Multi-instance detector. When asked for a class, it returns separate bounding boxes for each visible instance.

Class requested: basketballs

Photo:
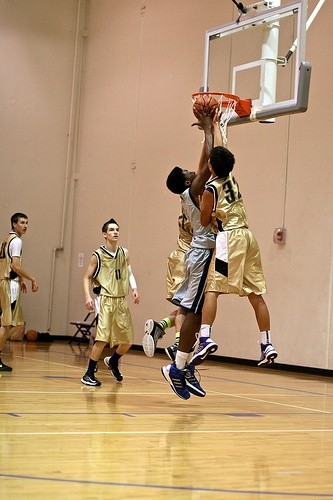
[192,96,220,121]
[27,329,38,342]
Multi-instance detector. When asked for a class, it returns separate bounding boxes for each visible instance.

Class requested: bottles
[89,333,94,347]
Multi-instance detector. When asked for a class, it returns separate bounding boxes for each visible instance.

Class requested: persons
[188,110,277,366]
[81,218,140,386]
[142,207,193,362]
[161,107,224,400]
[0,213,39,372]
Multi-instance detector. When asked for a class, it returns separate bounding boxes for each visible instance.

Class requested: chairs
[68,312,98,346]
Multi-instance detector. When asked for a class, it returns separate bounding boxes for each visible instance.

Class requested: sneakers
[142,319,165,357]
[184,364,206,397]
[0,361,12,371]
[258,343,278,366]
[161,361,190,400]
[189,333,218,366]
[81,364,101,386]
[104,357,123,381]
[165,343,178,363]
